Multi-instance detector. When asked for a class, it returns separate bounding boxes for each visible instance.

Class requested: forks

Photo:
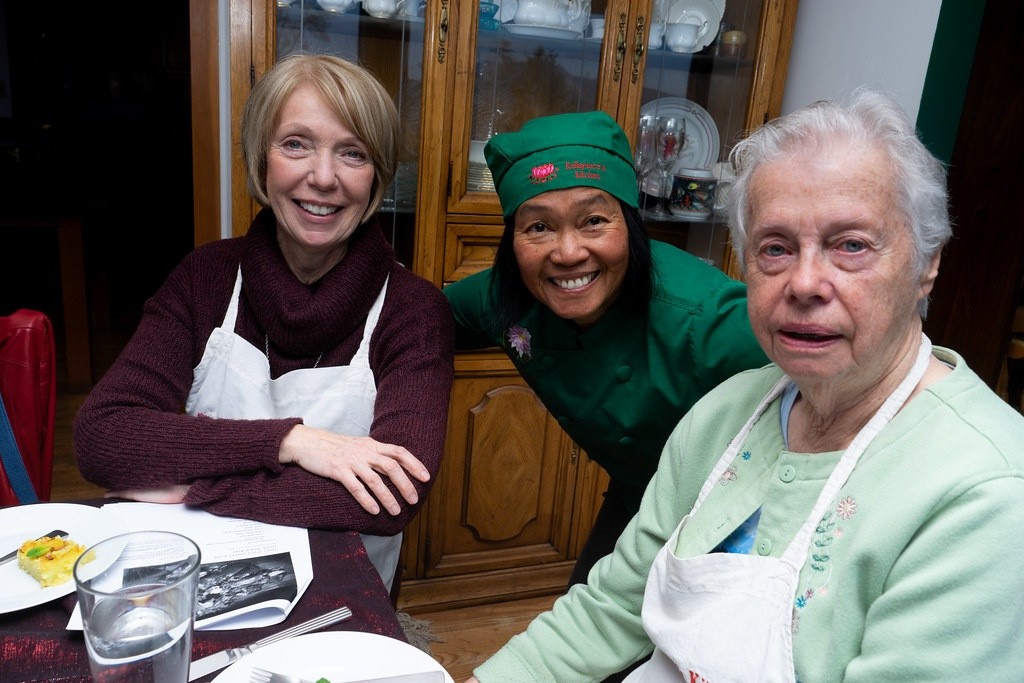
[249,665,445,683]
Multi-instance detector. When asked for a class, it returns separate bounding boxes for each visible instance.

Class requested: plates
[210,631,455,683]
[638,96,721,197]
[502,24,582,41]
[467,161,497,193]
[0,503,129,614]
[480,0,518,24]
[668,0,720,53]
[395,15,425,23]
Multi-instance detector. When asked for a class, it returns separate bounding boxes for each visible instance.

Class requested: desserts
[16,534,96,589]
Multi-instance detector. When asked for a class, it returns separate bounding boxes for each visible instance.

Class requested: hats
[483,110,641,217]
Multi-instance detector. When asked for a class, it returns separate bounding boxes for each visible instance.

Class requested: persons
[438,105,769,593]
[442,92,1024,682]
[69,52,455,598]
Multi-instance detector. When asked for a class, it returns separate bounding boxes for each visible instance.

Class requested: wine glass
[637,118,660,197]
[648,117,686,221]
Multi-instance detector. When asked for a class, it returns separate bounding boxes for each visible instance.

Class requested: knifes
[188,607,352,682]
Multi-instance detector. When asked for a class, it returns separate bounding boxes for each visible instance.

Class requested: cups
[647,23,666,50]
[668,167,732,222]
[361,0,403,19]
[469,141,488,163]
[316,0,355,15]
[397,0,426,16]
[72,531,202,683]
[276,0,297,7]
[667,20,711,53]
[590,19,604,38]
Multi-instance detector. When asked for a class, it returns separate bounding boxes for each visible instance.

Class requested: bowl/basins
[480,2,500,18]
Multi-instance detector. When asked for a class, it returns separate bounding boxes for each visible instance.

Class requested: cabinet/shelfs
[441,0,800,288]
[414,355,612,614]
[190,1,457,621]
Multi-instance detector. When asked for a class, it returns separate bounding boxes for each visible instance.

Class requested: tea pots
[514,0,581,29]
